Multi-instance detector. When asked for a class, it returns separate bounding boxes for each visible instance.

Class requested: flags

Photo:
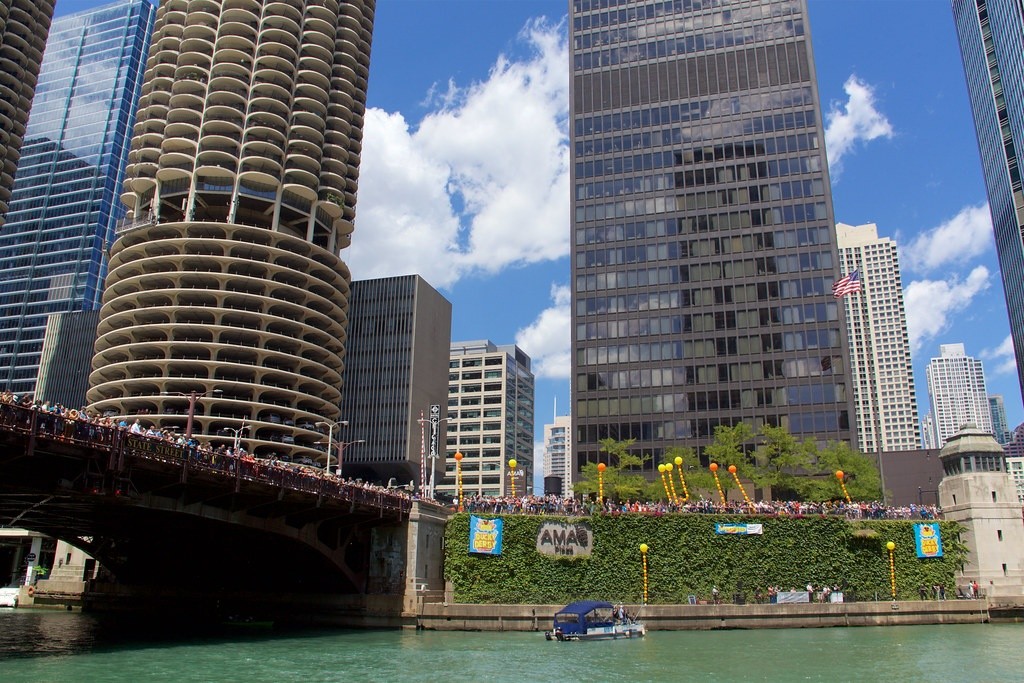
[832,269,861,299]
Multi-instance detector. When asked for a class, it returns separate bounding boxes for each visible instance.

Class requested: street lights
[314,440,366,479]
[224,425,252,455]
[160,390,223,445]
[417,417,452,502]
[314,421,349,478]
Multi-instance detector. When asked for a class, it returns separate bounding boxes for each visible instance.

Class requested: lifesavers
[28,585,34,595]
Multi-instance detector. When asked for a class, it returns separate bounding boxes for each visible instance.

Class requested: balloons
[887,541,896,597]
[508,459,517,495]
[455,452,462,511]
[729,465,750,502]
[598,463,605,499]
[709,462,725,502]
[640,543,648,601]
[658,456,689,501]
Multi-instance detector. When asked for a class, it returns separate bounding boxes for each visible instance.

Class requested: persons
[453,496,943,520]
[614,606,628,620]
[0,388,436,506]
[969,580,979,598]
[958,585,964,596]
[754,584,782,604]
[790,587,796,592]
[713,587,719,605]
[919,583,945,600]
[806,582,841,603]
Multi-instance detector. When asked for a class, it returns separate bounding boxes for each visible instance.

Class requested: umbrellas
[836,471,852,504]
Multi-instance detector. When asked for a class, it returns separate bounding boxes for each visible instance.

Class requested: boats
[545,601,647,642]
